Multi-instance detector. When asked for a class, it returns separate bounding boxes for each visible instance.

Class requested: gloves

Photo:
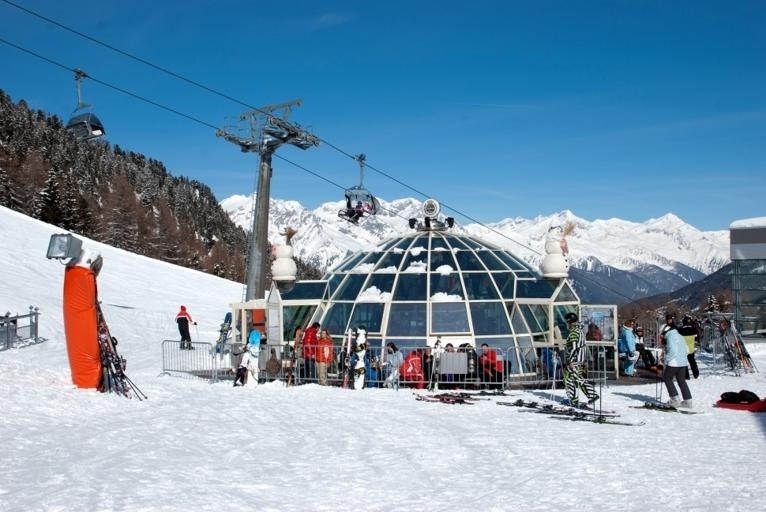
[194,322,197,325]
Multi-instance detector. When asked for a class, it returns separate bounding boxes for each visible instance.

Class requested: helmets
[683,318,690,325]
[635,327,644,336]
[565,313,578,323]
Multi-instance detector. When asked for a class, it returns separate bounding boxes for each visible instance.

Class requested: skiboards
[412,390,523,404]
[339,215,359,226]
[426,335,441,392]
[286,329,301,387]
[343,328,352,389]
[627,402,705,414]
[720,320,759,376]
[97,299,132,399]
[496,398,645,426]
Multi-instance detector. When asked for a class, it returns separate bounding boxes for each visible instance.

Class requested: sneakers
[680,399,692,406]
[667,395,679,405]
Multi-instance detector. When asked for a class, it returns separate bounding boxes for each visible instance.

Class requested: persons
[587,310,700,406]
[351,203,372,220]
[232,321,504,387]
[174,305,197,350]
[557,312,599,408]
[346,200,363,217]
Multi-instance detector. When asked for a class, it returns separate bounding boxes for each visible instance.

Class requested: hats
[181,306,186,310]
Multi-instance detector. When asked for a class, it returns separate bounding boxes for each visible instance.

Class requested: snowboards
[216,312,232,353]
[233,329,260,385]
[353,325,368,390]
